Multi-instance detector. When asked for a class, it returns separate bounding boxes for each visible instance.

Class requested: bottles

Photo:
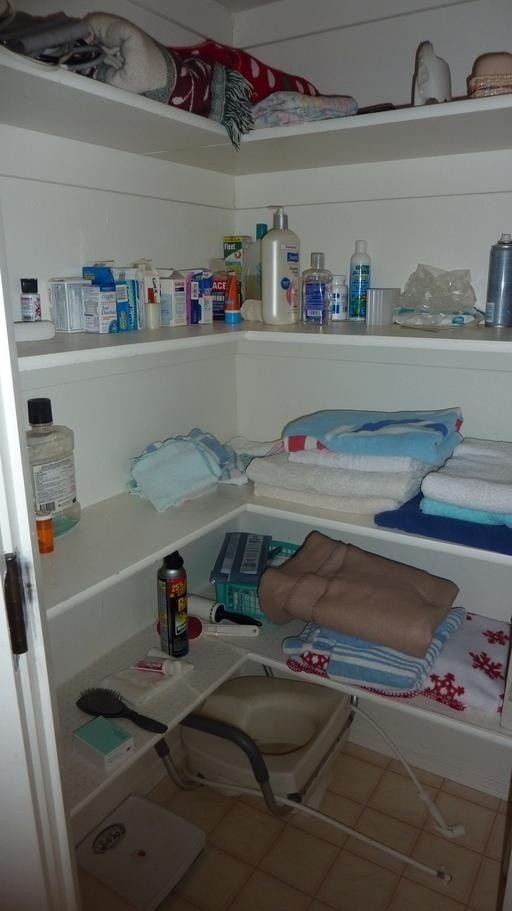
[347,240,372,322]
[22,398,81,538]
[298,252,333,327]
[260,206,304,327]
[483,233,512,328]
[157,550,189,658]
[331,275,349,322]
[241,223,269,300]
[34,512,56,553]
[20,279,41,321]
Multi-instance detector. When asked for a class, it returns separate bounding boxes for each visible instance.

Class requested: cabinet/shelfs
[0,0,512,857]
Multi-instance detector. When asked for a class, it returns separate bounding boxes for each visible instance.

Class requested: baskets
[215,541,301,622]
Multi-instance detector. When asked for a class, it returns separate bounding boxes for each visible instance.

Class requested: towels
[124,402,512,556]
[256,527,512,718]
